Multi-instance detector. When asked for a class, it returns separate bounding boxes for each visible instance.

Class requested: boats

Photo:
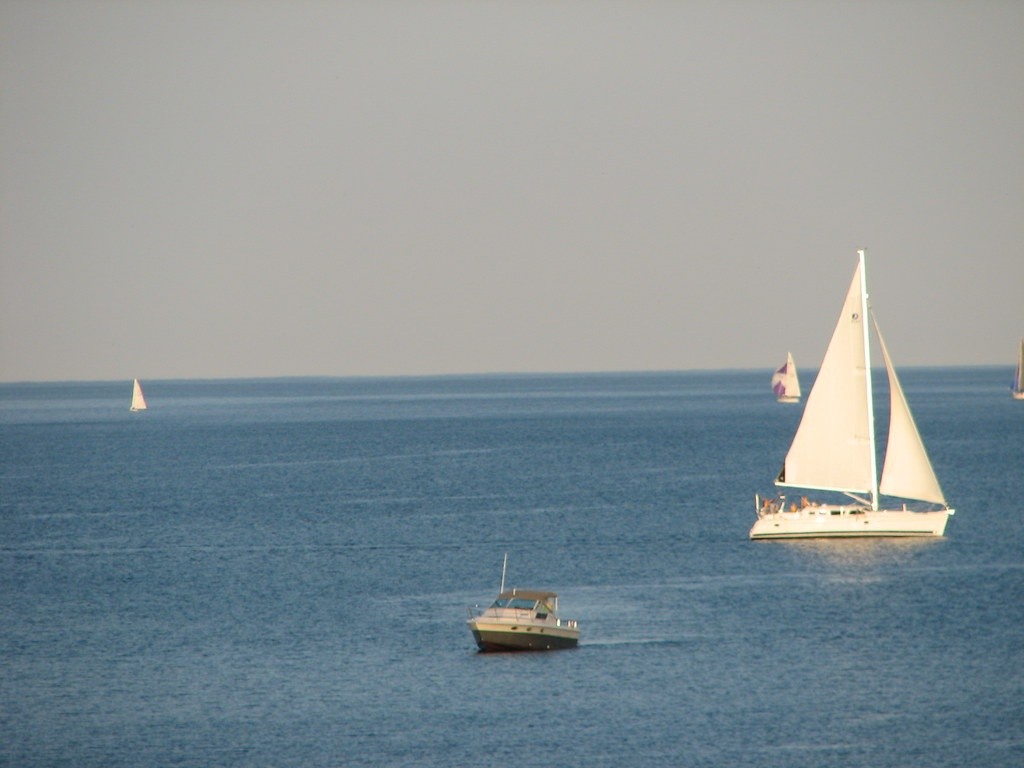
[464,551,582,652]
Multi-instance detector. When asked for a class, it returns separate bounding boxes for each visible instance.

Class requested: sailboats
[1011,337,1024,399]
[128,377,146,412]
[747,250,957,540]
[768,352,805,405]
